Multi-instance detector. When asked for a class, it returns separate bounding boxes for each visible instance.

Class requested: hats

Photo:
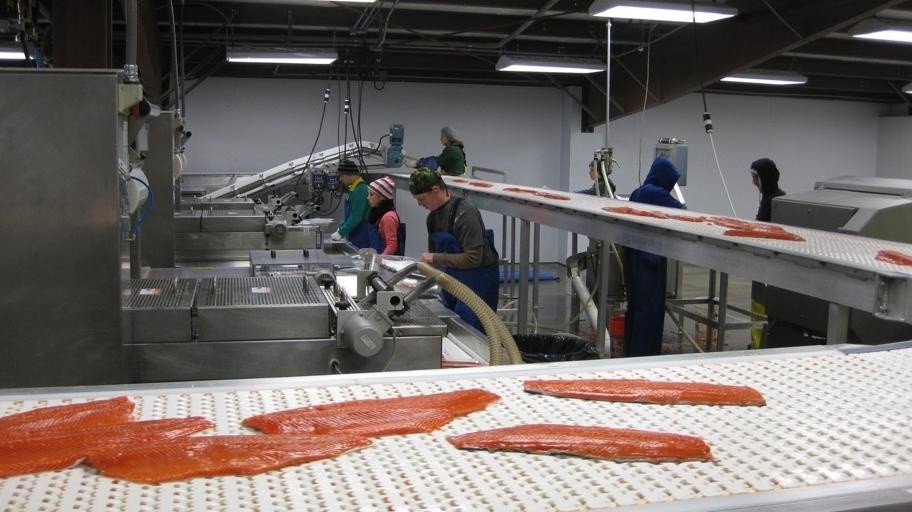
[408,167,441,193]
[441,126,456,137]
[336,159,359,174]
[368,175,397,201]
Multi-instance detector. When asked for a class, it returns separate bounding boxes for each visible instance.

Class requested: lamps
[722,71,808,85]
[590,0,738,25]
[851,20,912,44]
[224,50,339,66]
[496,58,607,75]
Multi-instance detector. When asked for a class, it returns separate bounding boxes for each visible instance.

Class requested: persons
[367,175,401,255]
[746,158,786,351]
[622,158,687,357]
[409,166,500,335]
[404,126,468,178]
[574,159,616,197]
[331,159,372,250]
